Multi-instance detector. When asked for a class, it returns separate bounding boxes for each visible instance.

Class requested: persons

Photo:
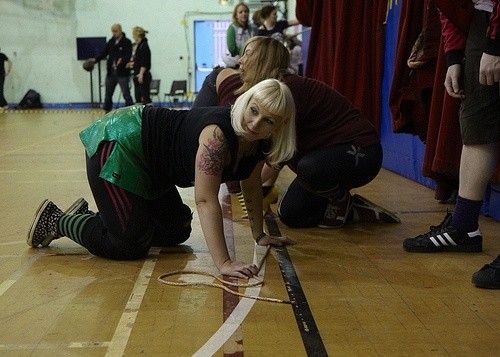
[402,0,500,288]
[226,3,303,70]
[193,66,248,106]
[25,78,299,280]
[234,36,402,227]
[89,23,134,113]
[0,52,12,108]
[129,26,152,105]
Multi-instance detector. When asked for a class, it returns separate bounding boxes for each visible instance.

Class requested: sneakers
[403,208,483,253]
[353,193,401,224]
[318,191,354,229]
[472,254,500,289]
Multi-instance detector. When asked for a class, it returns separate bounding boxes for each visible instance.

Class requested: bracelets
[255,233,266,243]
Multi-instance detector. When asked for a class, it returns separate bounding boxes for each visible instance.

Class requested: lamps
[218,0,233,5]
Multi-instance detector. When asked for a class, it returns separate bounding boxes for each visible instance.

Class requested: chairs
[150,79,161,102]
[164,80,188,103]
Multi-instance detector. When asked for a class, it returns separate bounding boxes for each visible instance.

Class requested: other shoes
[26,199,65,248]
[40,197,96,247]
